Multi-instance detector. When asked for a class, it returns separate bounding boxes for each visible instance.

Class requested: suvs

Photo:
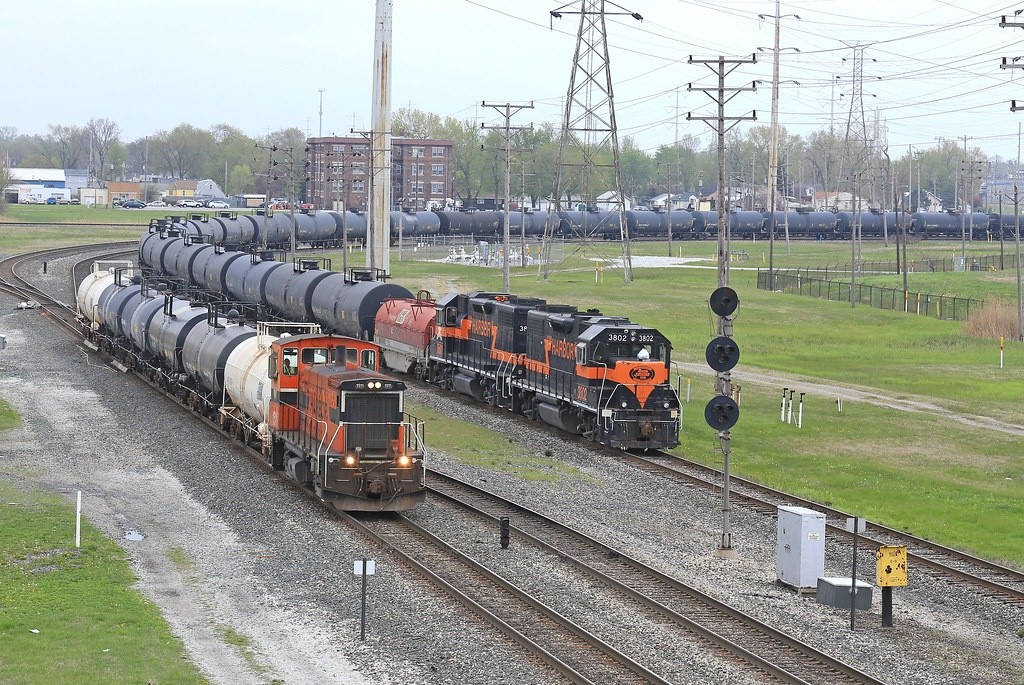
[112,197,125,206]
[47,198,56,205]
[177,200,203,208]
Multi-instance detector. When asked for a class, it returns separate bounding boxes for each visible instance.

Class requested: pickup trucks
[120,199,147,207]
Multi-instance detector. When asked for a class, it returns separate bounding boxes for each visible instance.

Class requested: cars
[71,199,80,205]
[259,200,291,209]
[60,199,68,205]
[122,201,144,209]
[146,200,166,208]
[36,198,45,205]
[198,199,231,208]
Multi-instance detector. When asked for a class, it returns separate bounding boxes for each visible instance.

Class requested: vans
[21,196,36,204]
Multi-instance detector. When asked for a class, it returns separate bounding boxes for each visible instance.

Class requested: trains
[138,208,1024,452]
[75,256,429,514]
